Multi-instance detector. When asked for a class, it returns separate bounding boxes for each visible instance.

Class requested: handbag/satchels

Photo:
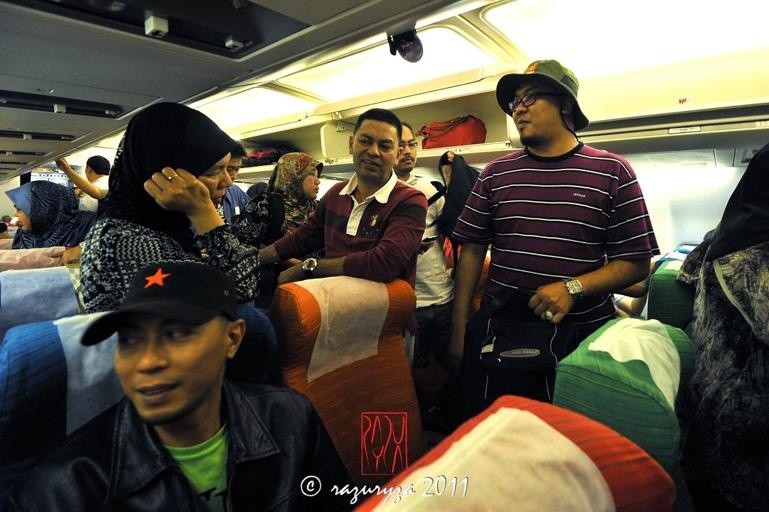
[416,115,487,149]
[449,290,557,418]
[243,148,279,167]
[435,151,481,267]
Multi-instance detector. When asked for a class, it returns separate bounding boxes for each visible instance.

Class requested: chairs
[0,222,765,512]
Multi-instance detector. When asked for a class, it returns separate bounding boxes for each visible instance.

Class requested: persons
[0,60,769,512]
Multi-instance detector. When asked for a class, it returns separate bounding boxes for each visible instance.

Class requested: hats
[80,263,241,346]
[5,183,31,216]
[496,61,588,130]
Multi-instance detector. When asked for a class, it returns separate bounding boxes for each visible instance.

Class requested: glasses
[508,90,560,112]
